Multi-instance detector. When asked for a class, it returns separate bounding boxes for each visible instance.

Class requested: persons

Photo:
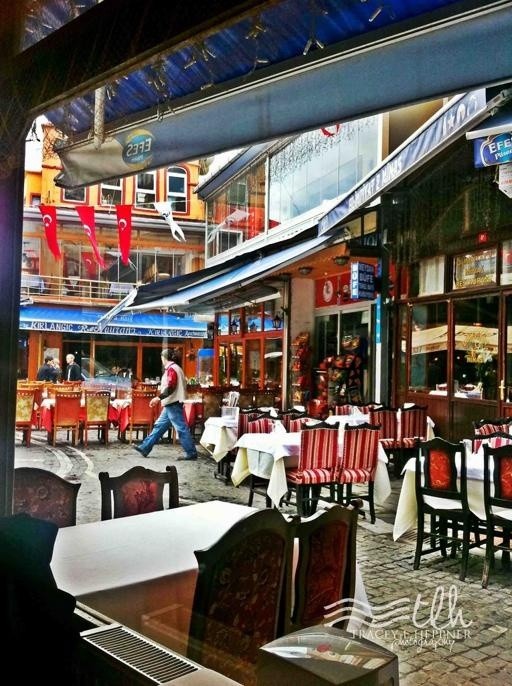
[59,353,81,382]
[35,355,59,381]
[132,348,199,460]
[107,364,133,379]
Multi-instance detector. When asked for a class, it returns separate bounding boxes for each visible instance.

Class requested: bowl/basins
[463,387,473,391]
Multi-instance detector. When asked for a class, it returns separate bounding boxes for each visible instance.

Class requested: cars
[80,357,131,388]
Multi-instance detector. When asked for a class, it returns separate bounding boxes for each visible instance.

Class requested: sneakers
[178,455,197,460]
[132,444,147,457]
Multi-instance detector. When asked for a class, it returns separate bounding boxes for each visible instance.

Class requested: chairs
[287,498,362,630]
[187,507,300,686]
[471,432,512,547]
[431,374,487,401]
[414,436,495,582]
[472,416,511,435]
[8,377,430,525]
[481,444,511,588]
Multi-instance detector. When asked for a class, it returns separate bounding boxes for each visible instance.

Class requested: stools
[257,625,399,686]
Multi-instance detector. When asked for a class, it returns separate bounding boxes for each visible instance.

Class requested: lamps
[297,265,317,275]
[230,308,242,335]
[268,305,290,329]
[247,302,260,332]
[331,255,350,265]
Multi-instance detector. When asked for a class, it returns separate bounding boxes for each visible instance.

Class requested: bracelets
[155,394,162,402]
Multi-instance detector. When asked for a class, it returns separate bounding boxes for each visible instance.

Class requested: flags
[74,204,109,270]
[40,205,64,262]
[205,202,266,245]
[63,253,104,298]
[113,203,137,270]
[152,200,188,243]
